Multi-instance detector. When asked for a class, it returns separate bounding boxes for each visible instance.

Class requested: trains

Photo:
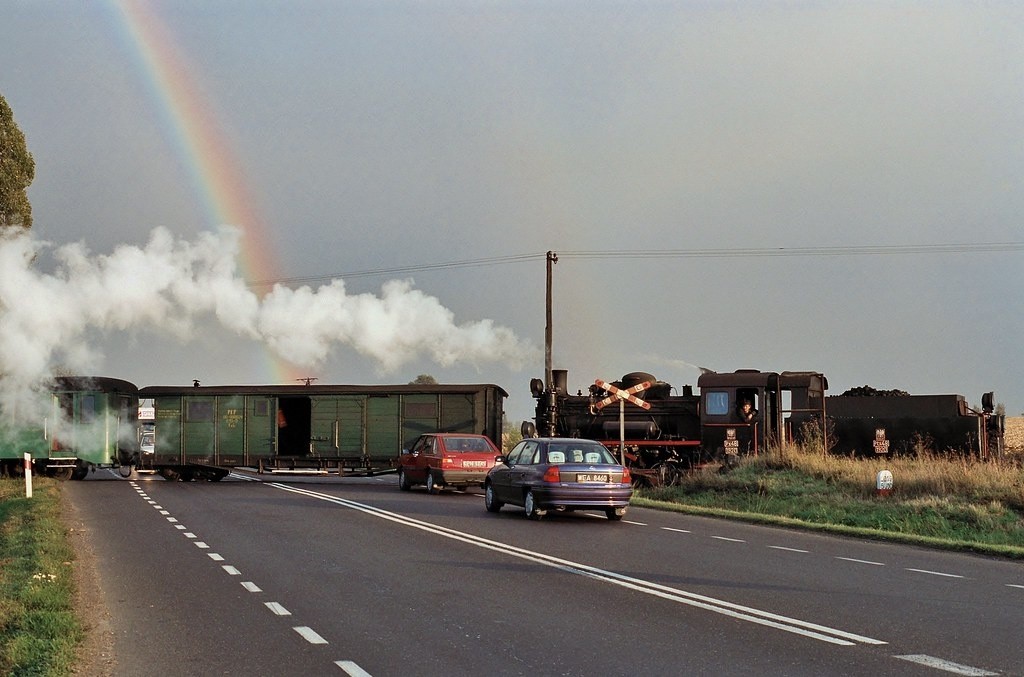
[1,359,1006,484]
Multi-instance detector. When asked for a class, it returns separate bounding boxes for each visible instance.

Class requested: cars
[136,432,156,472]
[483,437,634,521]
[396,432,504,495]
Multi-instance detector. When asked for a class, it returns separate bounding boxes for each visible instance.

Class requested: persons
[738,399,757,421]
